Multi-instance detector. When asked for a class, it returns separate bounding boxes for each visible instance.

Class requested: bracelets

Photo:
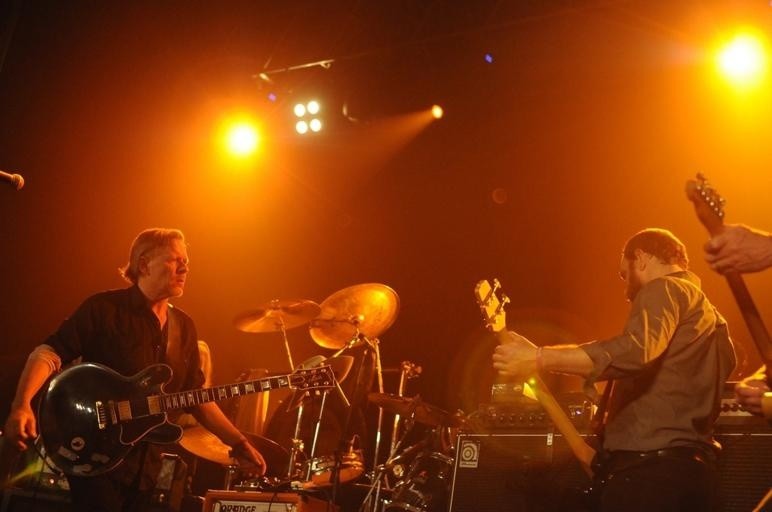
[535,345,544,371]
[227,433,250,459]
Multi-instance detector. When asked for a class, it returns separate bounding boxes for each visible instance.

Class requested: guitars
[475,278,605,511]
[686,171,772,423]
[36,362,336,477]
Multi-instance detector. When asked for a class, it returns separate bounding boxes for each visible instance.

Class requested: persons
[702,221,772,420]
[491,226,738,512]
[2,225,267,511]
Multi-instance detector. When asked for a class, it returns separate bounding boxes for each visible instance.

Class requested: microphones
[0,171,25,191]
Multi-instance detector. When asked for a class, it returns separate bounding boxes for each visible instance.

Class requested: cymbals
[309,283,400,350]
[178,426,241,466]
[241,430,290,473]
[367,392,462,429]
[234,298,322,334]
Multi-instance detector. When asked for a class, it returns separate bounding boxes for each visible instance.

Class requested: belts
[640,446,704,463]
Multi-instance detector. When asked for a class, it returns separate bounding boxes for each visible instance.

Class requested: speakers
[704,380,772,512]
[447,431,599,512]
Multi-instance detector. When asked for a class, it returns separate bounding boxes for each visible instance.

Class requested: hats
[286,354,355,413]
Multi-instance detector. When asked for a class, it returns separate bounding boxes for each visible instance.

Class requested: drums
[383,451,454,512]
[289,434,365,493]
